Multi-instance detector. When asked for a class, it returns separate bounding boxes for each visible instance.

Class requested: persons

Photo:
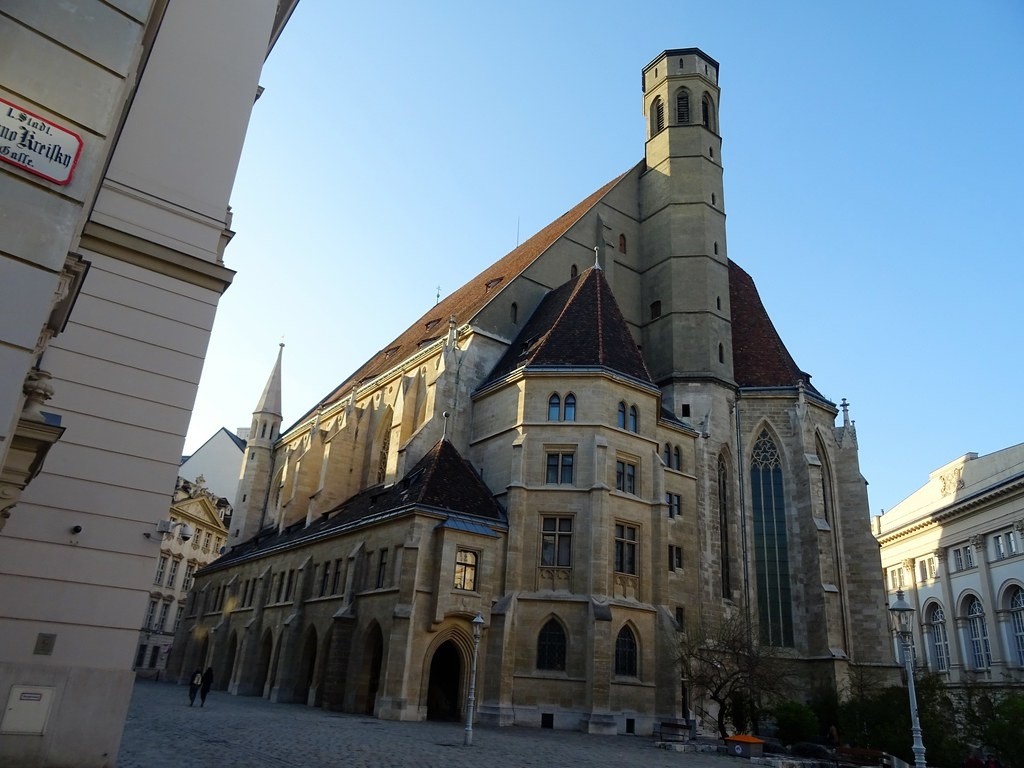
[188,667,202,706]
[962,751,1017,768]
[253,661,267,697]
[200,667,214,707]
[827,726,838,752]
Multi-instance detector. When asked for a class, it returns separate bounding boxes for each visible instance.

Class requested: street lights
[888,585,929,768]
[460,611,486,746]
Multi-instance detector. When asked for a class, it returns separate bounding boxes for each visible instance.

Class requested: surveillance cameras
[181,527,193,541]
